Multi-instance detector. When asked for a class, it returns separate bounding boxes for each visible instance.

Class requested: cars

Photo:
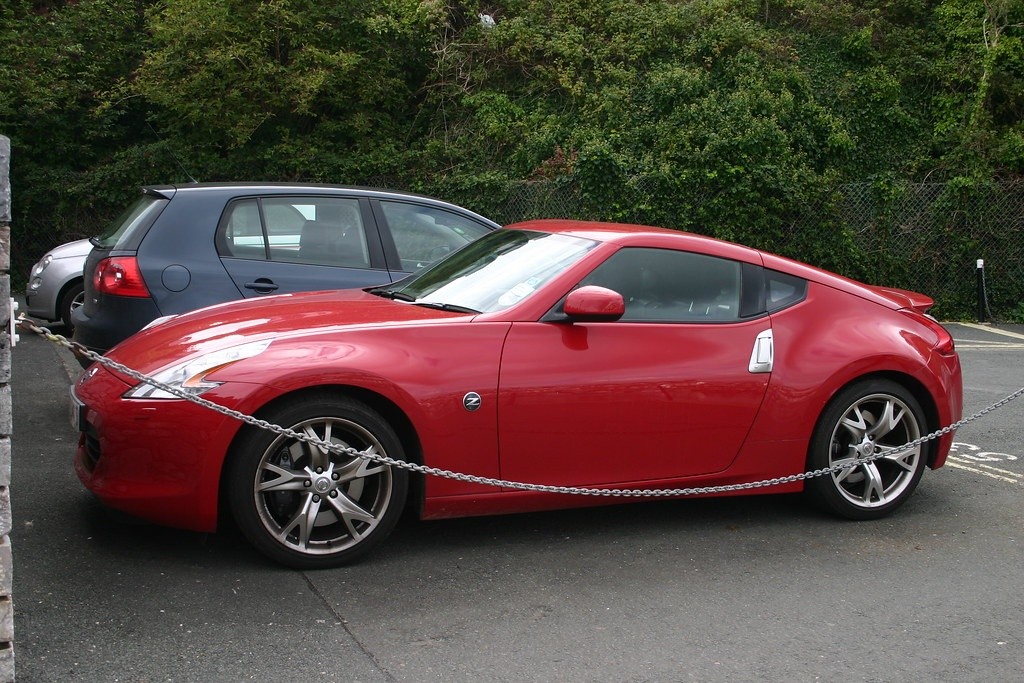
[69,119,504,369]
[68,220,965,569]
[27,202,319,329]
